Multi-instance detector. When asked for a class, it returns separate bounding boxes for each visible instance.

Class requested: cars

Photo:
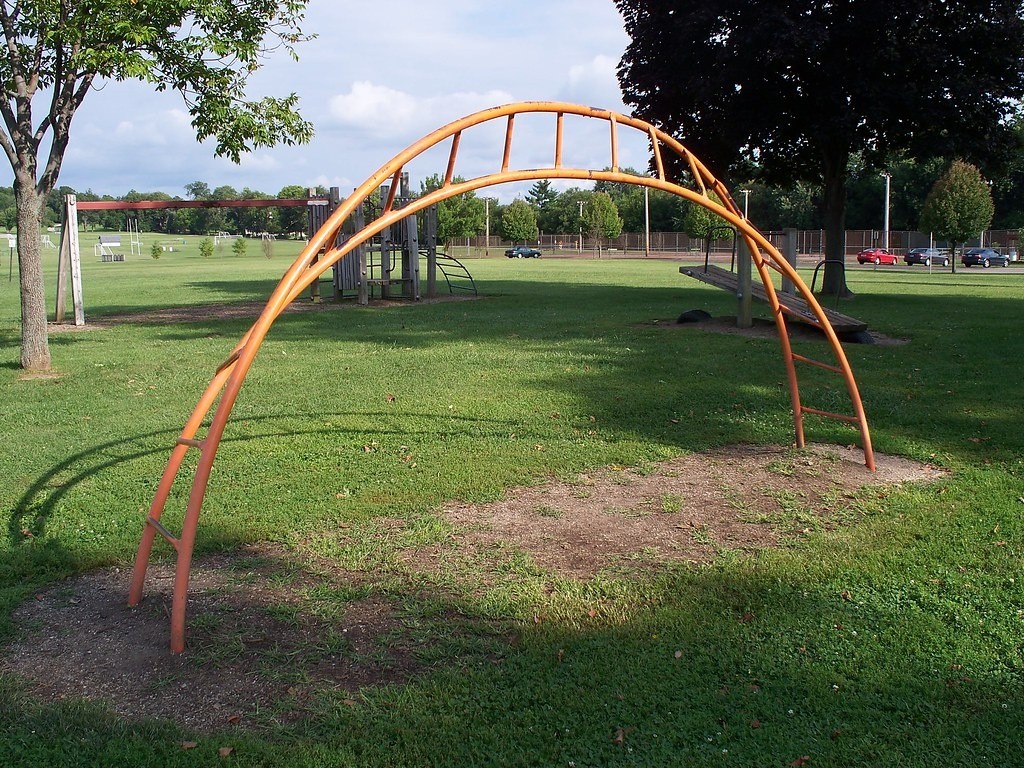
[505,246,541,258]
[961,248,1010,268]
[857,247,898,265]
[904,248,950,267]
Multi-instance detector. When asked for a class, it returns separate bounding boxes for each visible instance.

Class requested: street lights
[483,197,494,255]
[577,200,588,253]
[879,173,892,253]
[739,189,753,219]
